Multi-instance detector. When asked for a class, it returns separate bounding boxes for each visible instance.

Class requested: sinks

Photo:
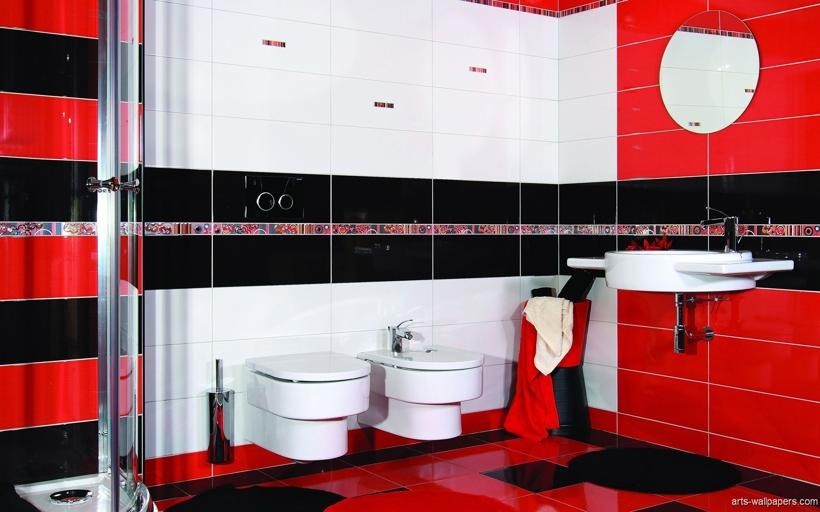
[358,348,485,440]
[604,250,757,291]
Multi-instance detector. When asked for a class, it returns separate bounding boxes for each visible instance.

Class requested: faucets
[697,205,738,251]
[387,319,411,355]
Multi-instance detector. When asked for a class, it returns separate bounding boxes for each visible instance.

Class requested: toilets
[245,352,371,461]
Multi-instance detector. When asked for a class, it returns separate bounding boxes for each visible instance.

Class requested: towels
[501,292,574,442]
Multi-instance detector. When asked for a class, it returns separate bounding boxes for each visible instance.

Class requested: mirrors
[655,8,760,137]
